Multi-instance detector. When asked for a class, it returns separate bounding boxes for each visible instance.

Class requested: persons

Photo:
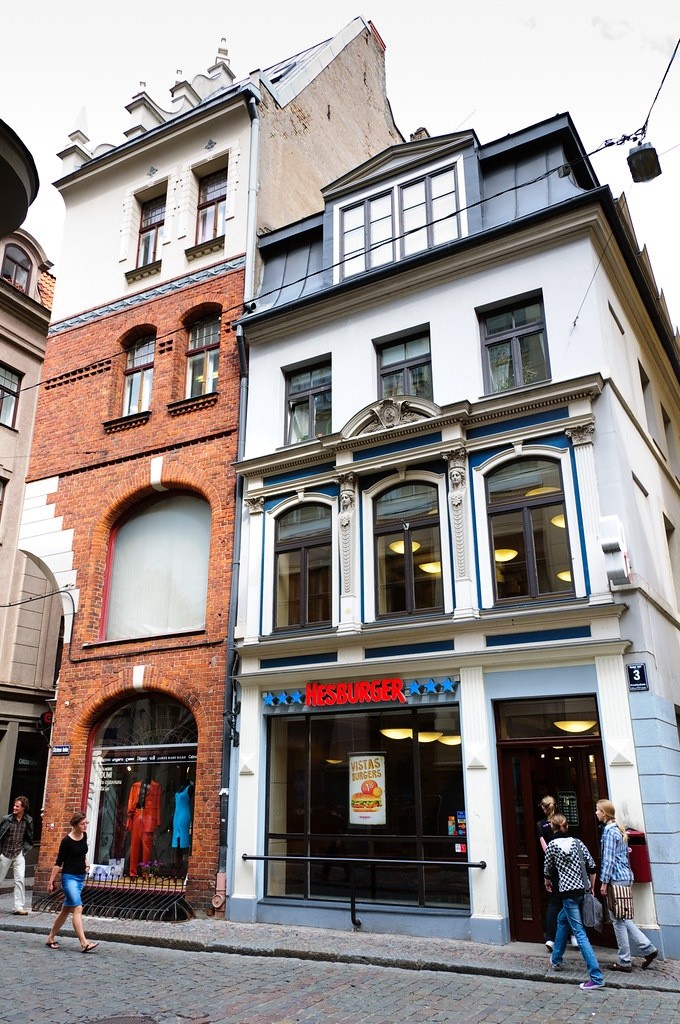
[544,814,606,989]
[595,800,658,973]
[171,778,194,879]
[126,774,162,882]
[537,796,579,952]
[0,796,33,916]
[45,813,99,953]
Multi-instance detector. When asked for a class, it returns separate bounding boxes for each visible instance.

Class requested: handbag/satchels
[584,893,603,930]
[608,883,634,919]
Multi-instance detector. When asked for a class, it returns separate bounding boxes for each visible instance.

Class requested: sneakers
[549,956,561,970]
[580,981,604,990]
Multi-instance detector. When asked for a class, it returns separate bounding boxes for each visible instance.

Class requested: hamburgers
[351,792,384,812]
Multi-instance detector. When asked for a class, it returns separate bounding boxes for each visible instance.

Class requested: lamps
[388,515,574,582]
[438,734,462,745]
[380,726,414,740]
[408,731,443,742]
[553,720,597,733]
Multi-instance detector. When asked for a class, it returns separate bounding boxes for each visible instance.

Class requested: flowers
[140,859,162,879]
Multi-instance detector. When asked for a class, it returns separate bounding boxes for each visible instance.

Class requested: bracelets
[86,866,90,868]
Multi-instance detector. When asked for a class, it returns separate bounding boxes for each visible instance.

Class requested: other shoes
[545,940,555,951]
[607,962,632,972]
[570,937,579,947]
[14,909,28,915]
[641,951,658,968]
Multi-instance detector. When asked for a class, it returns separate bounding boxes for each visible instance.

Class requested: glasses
[78,821,89,825]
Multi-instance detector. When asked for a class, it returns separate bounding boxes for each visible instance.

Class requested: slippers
[81,942,99,953]
[46,942,56,947]
